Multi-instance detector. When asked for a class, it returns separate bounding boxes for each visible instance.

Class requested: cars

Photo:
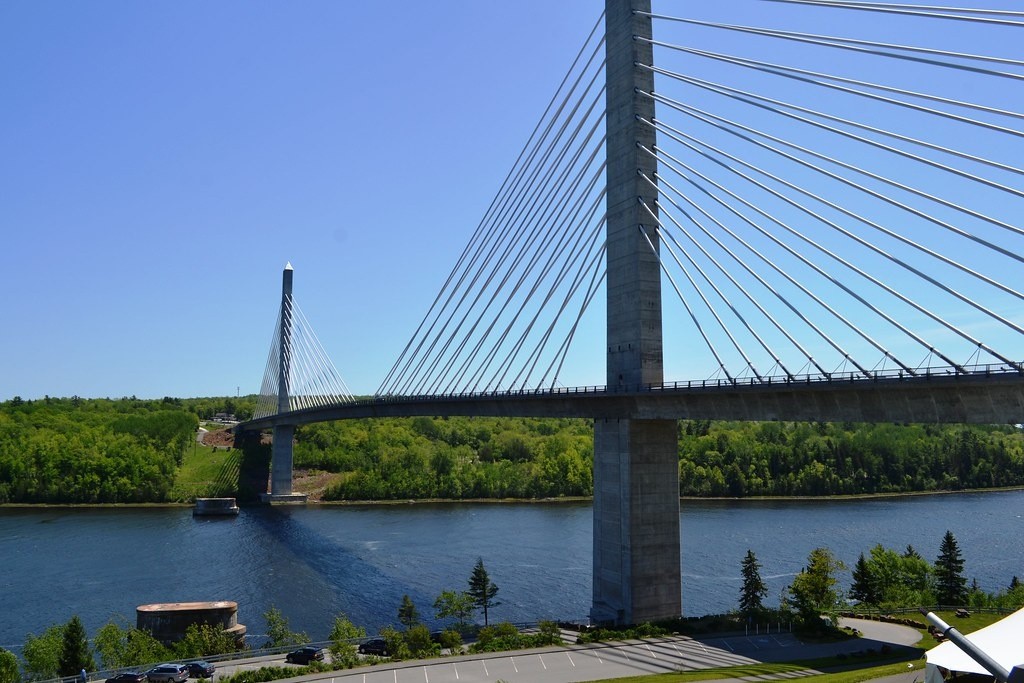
[430,630,447,644]
[358,637,395,657]
[178,661,215,678]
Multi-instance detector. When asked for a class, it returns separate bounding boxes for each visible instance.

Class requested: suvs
[285,646,324,665]
[105,672,149,683]
[141,664,191,683]
